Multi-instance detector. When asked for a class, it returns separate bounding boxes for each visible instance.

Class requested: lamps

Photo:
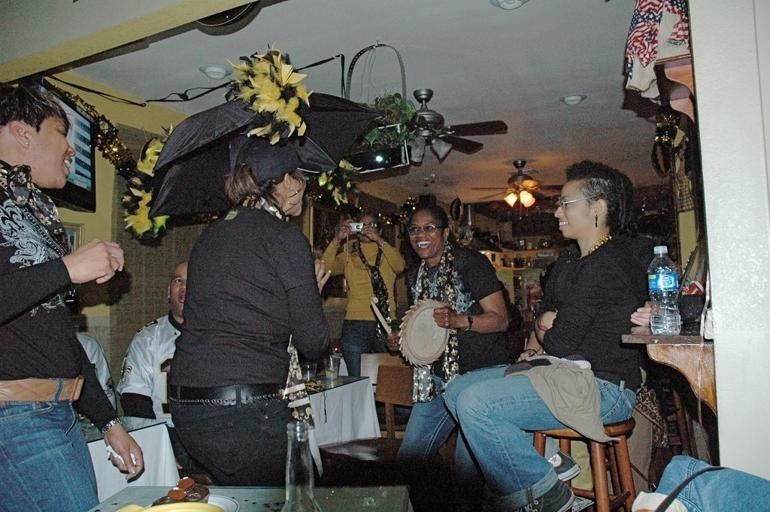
[504,186,536,208]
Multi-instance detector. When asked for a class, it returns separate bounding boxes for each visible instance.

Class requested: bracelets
[100,419,121,434]
[379,240,386,248]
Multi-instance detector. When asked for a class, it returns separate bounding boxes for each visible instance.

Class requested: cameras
[348,222,363,232]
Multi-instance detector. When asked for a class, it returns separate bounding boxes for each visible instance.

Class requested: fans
[471,159,564,200]
[406,89,508,166]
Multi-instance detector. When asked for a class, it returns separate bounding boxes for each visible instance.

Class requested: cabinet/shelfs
[621,54,717,469]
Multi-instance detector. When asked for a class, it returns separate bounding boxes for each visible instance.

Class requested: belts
[175,384,285,408]
[0,375,84,403]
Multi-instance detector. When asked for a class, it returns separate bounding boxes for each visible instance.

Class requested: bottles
[281,421,326,512]
[646,246,681,337]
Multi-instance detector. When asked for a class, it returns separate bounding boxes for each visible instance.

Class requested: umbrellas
[149,87,385,220]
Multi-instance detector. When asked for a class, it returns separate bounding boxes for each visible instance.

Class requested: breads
[168,476,211,504]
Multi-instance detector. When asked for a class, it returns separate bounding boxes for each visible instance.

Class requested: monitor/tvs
[342,123,409,174]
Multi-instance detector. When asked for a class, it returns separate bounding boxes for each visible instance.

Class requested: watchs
[466,314,473,333]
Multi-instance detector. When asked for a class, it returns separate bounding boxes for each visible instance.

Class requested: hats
[237,131,322,184]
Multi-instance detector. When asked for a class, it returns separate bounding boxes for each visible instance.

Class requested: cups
[300,362,317,381]
[324,356,340,382]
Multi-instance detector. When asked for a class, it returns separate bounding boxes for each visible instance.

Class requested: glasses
[557,196,588,212]
[406,223,449,235]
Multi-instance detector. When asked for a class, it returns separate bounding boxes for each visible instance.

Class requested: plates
[144,494,239,512]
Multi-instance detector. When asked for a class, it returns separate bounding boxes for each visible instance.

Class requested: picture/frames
[60,221,85,256]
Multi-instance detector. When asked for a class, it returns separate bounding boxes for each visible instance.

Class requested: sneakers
[504,480,576,511]
[547,450,581,481]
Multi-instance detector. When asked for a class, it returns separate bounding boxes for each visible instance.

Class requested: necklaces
[585,233,612,254]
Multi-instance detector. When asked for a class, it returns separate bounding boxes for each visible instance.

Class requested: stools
[532,417,637,512]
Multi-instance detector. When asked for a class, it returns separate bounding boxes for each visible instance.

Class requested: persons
[1,83,145,510]
[119,263,189,434]
[324,213,408,373]
[444,161,651,512]
[386,203,522,481]
[167,135,331,489]
[76,331,115,411]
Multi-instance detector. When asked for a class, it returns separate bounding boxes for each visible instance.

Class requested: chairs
[297,352,460,512]
